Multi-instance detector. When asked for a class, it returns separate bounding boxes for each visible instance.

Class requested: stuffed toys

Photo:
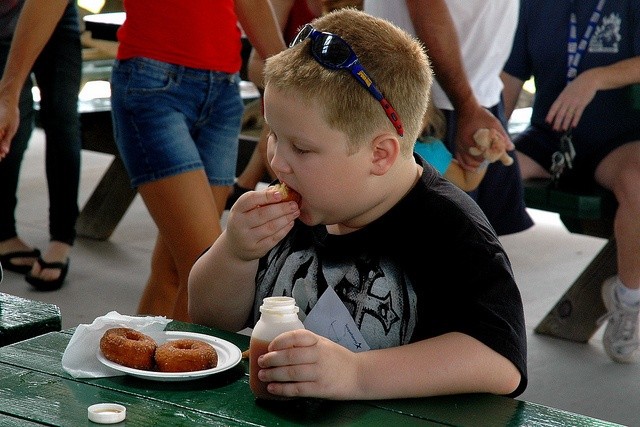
[469,127,514,167]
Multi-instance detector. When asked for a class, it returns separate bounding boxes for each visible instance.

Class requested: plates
[94,329,244,382]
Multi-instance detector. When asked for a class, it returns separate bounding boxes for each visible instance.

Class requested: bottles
[250,297,305,401]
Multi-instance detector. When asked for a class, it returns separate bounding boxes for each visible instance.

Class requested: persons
[1,0,290,321]
[363,0,538,238]
[409,91,515,195]
[0,0,82,292]
[187,6,528,403]
[499,0,640,364]
[223,0,365,215]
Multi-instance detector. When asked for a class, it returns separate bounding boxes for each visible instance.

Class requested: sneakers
[596,276,640,363]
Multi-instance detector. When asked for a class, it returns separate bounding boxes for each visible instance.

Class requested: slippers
[0,249,41,272]
[26,256,69,291]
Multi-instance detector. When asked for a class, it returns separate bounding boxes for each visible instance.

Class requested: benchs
[33,101,619,343]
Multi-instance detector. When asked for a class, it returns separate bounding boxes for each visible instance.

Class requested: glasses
[291,24,403,137]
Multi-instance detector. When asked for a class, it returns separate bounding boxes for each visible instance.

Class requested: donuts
[100,327,157,371]
[154,339,218,372]
